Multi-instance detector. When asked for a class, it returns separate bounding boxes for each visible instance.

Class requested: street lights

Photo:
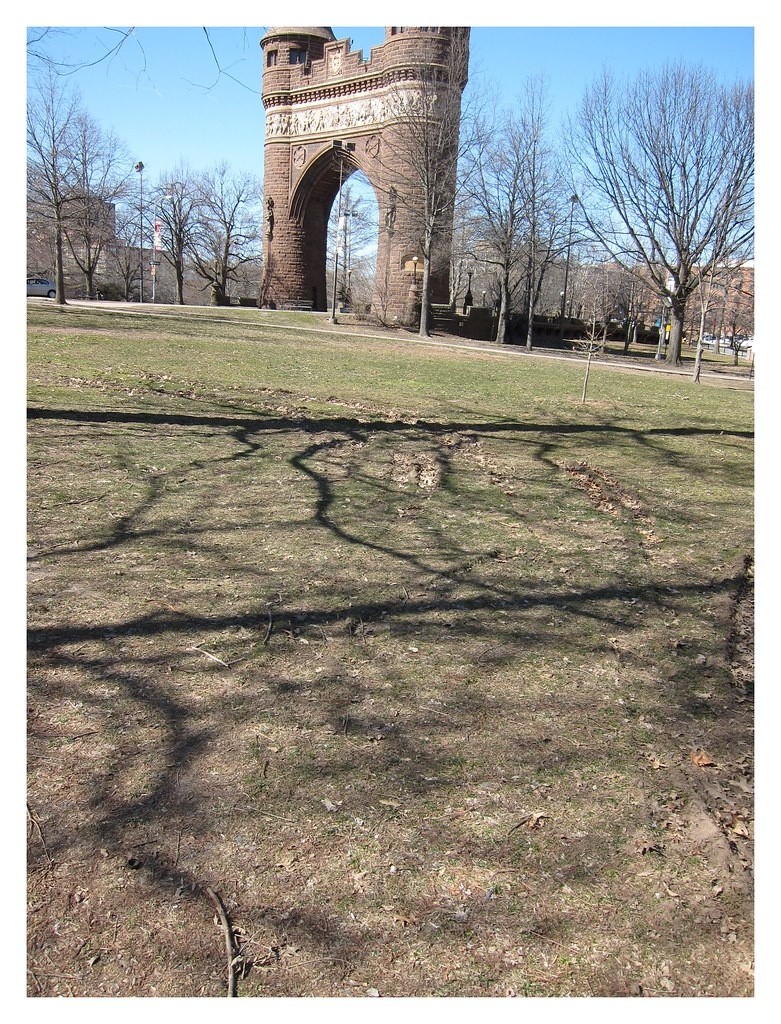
[344,210,360,304]
[134,161,146,304]
[556,194,581,349]
[328,139,356,326]
[151,195,173,303]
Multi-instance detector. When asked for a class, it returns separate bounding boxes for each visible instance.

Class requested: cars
[27,277,57,298]
